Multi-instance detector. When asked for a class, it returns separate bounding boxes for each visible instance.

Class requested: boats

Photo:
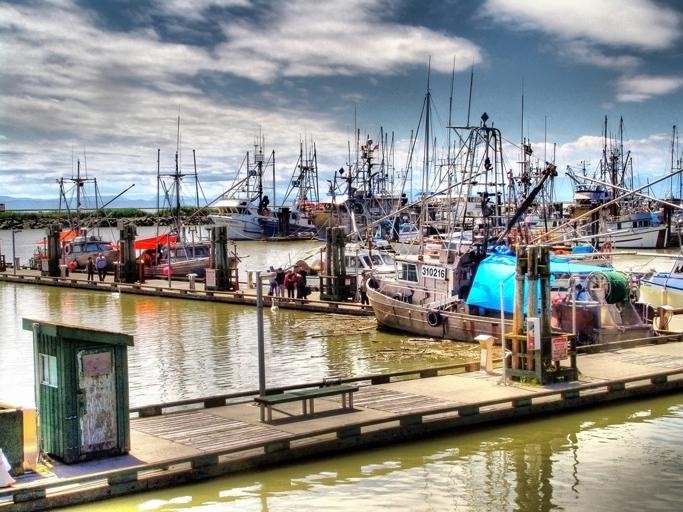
[364,112,654,351]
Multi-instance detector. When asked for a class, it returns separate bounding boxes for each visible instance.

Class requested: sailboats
[27,139,134,271]
[119,115,255,283]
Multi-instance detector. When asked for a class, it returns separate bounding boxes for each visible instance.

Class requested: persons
[86,257,95,280]
[96,252,107,281]
[269,265,307,300]
[360,271,369,308]
